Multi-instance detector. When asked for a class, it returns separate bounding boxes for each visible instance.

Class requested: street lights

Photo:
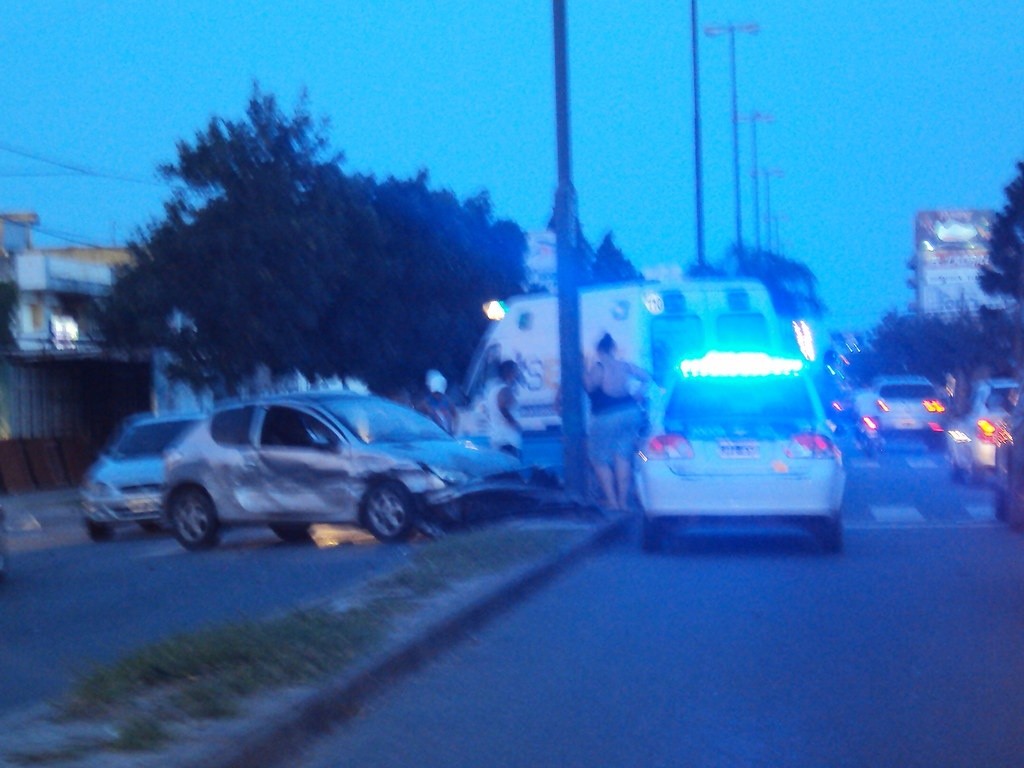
[703,20,760,242]
[732,105,785,254]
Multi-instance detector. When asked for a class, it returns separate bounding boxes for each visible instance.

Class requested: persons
[413,366,460,437]
[579,332,652,516]
[488,358,526,460]
[854,380,883,451]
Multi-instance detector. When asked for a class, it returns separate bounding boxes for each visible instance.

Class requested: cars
[163,391,523,553]
[993,389,1024,529]
[855,372,946,443]
[941,376,1024,484]
[80,409,211,540]
[632,346,846,556]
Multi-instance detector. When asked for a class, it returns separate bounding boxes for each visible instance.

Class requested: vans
[443,279,786,495]
